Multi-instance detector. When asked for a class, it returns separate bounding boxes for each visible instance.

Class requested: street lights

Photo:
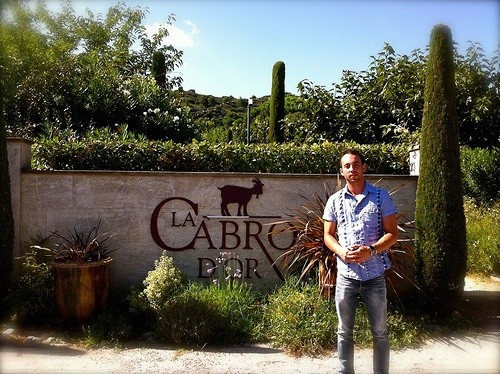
[246,98,253,145]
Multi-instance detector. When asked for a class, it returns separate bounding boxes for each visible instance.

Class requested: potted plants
[265,167,422,306]
[44,217,112,324]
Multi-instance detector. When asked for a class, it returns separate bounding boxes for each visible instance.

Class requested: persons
[321,149,399,374]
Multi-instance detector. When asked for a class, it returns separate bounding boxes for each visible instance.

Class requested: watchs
[369,246,377,257]
